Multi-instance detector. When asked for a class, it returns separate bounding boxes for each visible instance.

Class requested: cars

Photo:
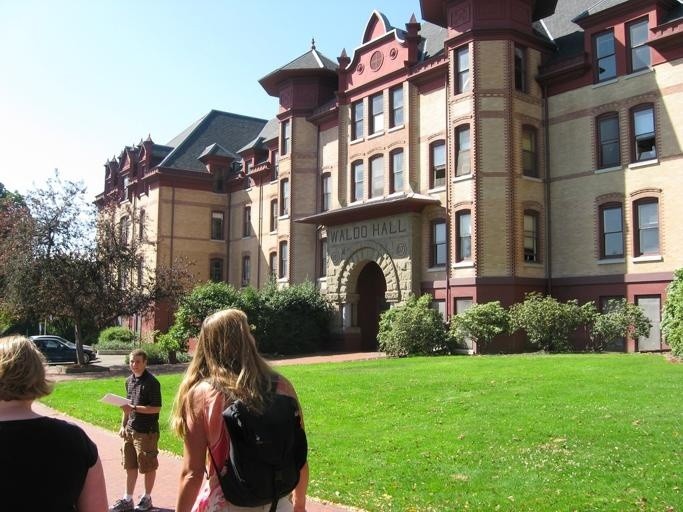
[28,335,97,365]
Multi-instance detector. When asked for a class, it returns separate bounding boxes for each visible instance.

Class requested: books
[97,392,131,407]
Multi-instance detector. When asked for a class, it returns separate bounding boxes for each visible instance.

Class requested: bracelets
[130,405,136,412]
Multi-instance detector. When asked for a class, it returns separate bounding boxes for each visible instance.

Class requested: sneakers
[134,495,153,512]
[108,498,134,511]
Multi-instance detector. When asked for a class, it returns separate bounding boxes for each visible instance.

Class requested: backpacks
[202,370,308,507]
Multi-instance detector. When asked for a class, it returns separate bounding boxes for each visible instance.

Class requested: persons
[165,307,309,511]
[102,348,161,512]
[0,333,110,511]
